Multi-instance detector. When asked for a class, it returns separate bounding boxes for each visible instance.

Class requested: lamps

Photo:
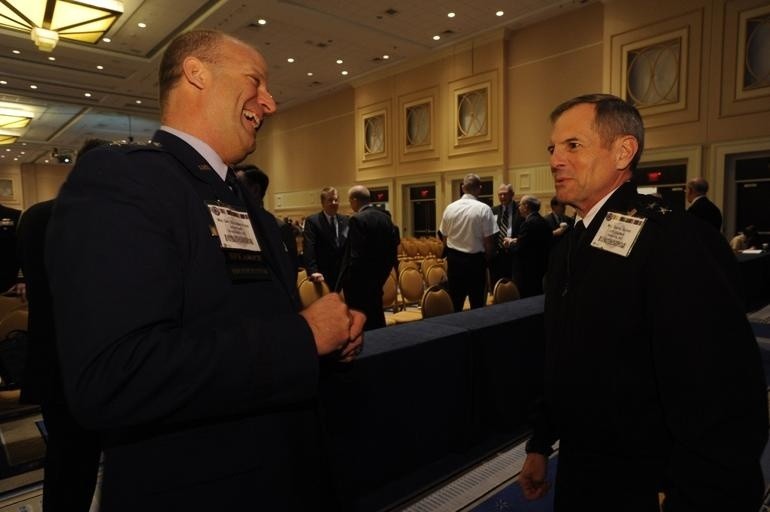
[0,1,124,53]
[0,108,33,145]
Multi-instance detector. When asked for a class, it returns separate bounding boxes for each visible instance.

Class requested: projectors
[51,153,73,166]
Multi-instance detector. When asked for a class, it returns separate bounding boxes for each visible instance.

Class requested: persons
[729,224,758,250]
[683,176,722,235]
[302,186,348,296]
[384,211,401,267]
[543,196,571,236]
[283,215,306,234]
[340,185,395,331]
[39,27,366,511]
[438,173,501,313]
[14,137,111,512]
[503,195,554,299]
[507,92,767,510]
[486,183,526,296]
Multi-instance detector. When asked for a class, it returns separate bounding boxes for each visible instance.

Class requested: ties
[498,207,508,248]
[330,216,336,241]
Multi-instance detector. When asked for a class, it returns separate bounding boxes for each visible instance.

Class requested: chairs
[295,235,523,320]
